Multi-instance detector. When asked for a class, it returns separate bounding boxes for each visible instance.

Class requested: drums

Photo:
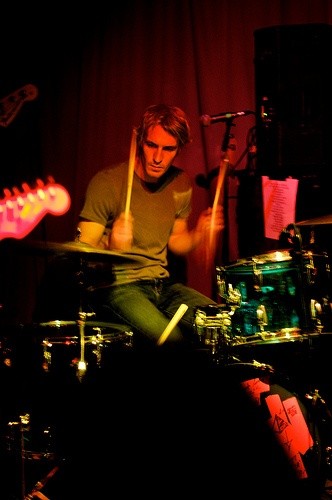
[8,308,134,379]
[0,419,55,463]
[216,249,332,347]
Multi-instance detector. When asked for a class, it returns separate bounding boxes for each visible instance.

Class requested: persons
[76,103,221,352]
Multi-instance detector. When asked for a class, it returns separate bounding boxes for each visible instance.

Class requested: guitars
[1,177,71,239]
[0,84,38,127]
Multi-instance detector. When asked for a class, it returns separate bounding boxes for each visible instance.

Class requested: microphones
[201,110,251,126]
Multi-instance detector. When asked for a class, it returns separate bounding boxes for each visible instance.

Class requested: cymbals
[296,215,332,226]
[41,241,163,267]
